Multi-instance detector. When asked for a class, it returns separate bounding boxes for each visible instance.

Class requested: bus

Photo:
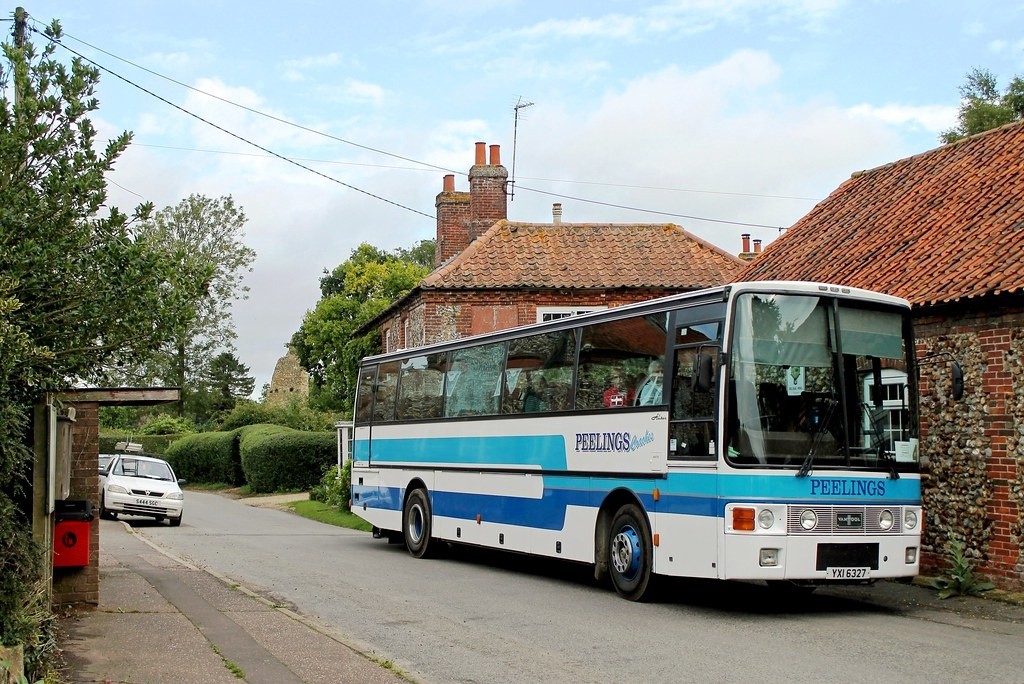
[351,280,964,602]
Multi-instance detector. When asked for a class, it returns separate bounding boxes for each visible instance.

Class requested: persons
[523,375,559,412]
[639,360,669,403]
[602,369,635,407]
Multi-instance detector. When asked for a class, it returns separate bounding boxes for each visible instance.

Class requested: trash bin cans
[53,498,94,568]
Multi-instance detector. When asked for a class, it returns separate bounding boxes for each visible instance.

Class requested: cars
[99,454,187,526]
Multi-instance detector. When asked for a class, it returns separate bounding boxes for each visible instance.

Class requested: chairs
[361,395,443,421]
[503,387,522,413]
